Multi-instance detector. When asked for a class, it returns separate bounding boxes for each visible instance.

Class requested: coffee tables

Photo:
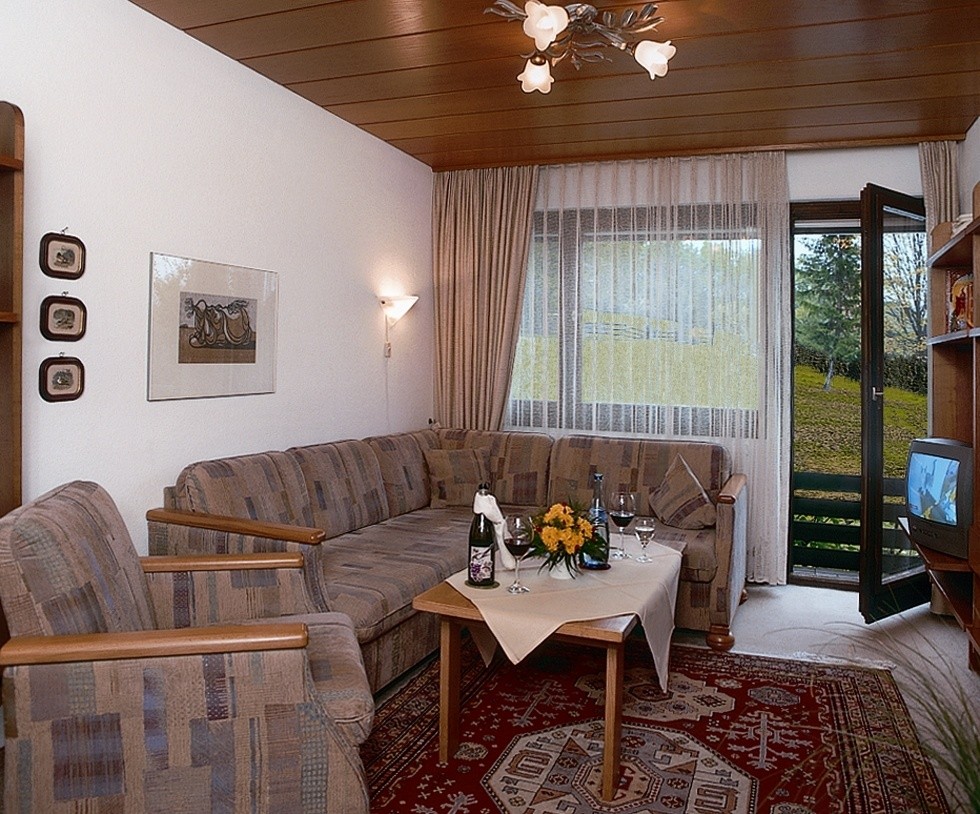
[412,534,688,803]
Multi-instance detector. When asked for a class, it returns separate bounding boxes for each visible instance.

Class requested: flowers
[520,494,610,580]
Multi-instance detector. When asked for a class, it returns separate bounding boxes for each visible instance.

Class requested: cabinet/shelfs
[895,180,980,678]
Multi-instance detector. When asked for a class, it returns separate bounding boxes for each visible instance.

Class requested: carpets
[359,630,952,814]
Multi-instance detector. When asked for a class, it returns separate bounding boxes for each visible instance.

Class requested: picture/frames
[38,357,85,403]
[40,233,87,280]
[40,295,86,342]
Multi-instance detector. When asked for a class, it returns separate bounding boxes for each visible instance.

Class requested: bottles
[581,473,609,569]
[467,482,496,586]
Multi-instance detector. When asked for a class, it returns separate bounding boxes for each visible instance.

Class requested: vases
[548,550,575,580]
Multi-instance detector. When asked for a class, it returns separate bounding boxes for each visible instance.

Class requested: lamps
[482,0,677,95]
[377,293,420,358]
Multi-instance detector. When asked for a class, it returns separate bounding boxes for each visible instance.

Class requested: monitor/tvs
[908,437,973,559]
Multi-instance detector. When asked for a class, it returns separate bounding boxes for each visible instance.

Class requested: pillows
[421,446,493,509]
[648,451,718,529]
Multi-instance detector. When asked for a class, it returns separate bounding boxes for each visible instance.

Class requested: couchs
[145,427,749,693]
[0,481,377,814]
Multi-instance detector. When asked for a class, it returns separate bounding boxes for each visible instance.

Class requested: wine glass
[503,513,536,593]
[634,518,655,563]
[609,492,637,559]
